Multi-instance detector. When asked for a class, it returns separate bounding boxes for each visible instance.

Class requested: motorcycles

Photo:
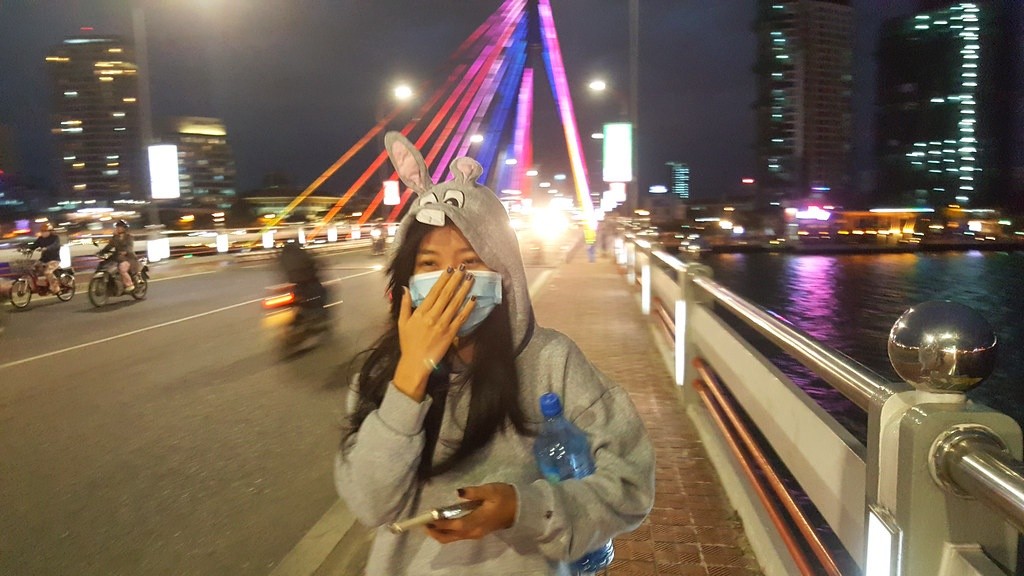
[7,246,77,309]
[88,240,151,309]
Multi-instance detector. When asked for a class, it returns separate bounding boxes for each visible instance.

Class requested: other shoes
[125,285,136,292]
[40,288,54,297]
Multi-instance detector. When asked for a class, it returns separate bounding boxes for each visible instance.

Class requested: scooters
[259,273,344,355]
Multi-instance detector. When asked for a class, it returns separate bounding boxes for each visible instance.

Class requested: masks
[408,270,503,338]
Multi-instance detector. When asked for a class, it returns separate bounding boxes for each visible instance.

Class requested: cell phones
[388,499,482,534]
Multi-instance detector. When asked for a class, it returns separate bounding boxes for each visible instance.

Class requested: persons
[278,242,328,322]
[98,220,140,293]
[19,222,62,296]
[330,131,657,576]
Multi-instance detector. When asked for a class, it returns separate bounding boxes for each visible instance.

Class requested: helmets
[115,220,130,228]
[40,223,53,231]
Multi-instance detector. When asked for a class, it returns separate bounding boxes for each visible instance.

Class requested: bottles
[533,393,615,572]
